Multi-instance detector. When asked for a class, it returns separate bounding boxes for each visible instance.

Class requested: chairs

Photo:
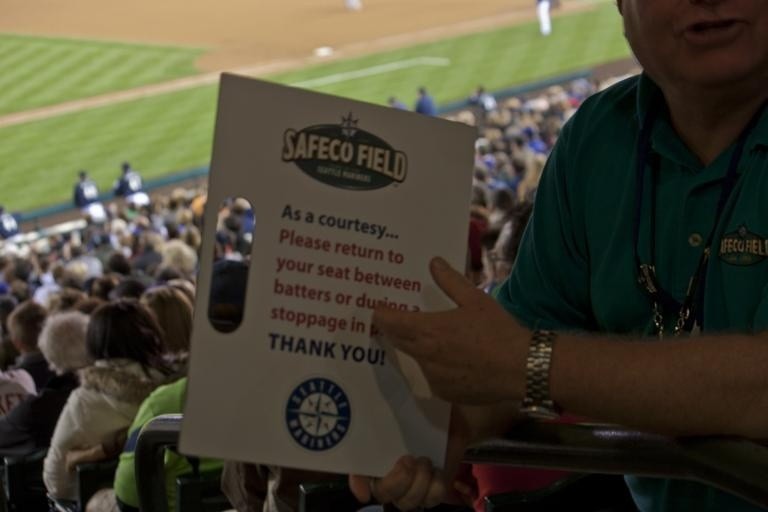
[2,446,373,511]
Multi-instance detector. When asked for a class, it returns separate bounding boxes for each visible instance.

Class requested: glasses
[486,250,511,263]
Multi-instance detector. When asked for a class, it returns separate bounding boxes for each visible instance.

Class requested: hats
[467,220,487,264]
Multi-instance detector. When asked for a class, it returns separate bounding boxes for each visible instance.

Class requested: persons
[349,0,767,512]
[535,0,552,36]
[0,72,636,512]
